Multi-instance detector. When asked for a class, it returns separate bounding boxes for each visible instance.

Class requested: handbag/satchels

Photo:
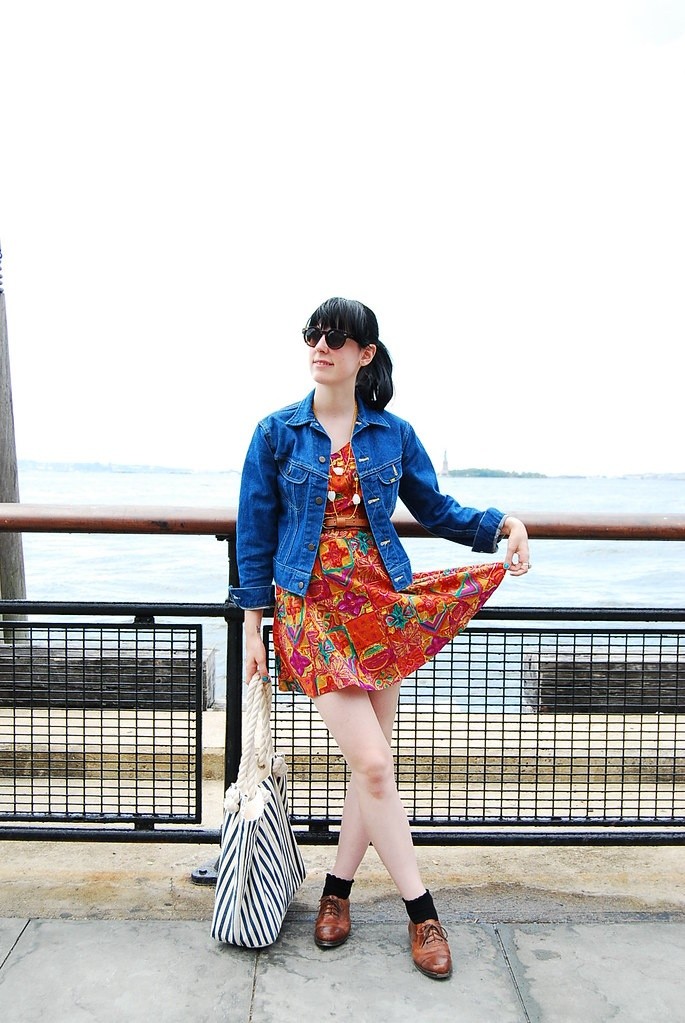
[208,670,305,950]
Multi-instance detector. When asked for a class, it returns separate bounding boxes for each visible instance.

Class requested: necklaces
[312,402,360,519]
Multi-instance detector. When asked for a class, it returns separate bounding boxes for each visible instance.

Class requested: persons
[228,297,531,980]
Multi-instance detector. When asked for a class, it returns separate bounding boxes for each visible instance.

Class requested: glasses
[302,325,364,351]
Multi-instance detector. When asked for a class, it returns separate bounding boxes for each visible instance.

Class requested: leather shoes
[406,920,454,980]
[313,896,352,949]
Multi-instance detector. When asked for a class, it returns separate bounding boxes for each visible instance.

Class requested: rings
[523,563,531,569]
